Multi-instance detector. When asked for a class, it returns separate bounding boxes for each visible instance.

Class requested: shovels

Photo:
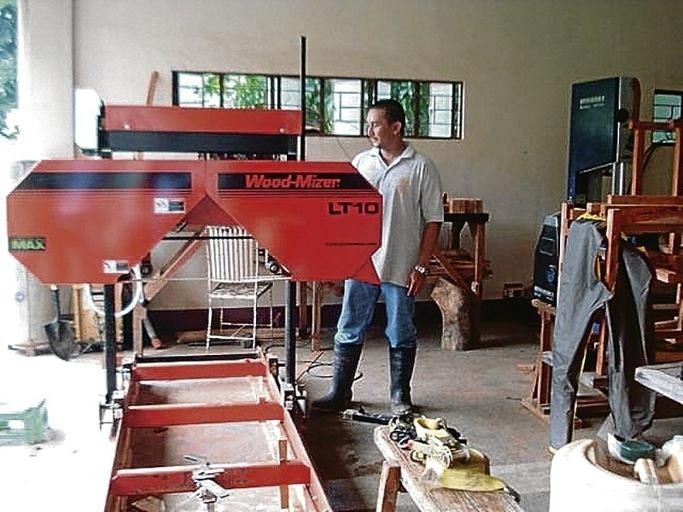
[42,284,76,361]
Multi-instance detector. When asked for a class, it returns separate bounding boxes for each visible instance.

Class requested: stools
[0,397,48,446]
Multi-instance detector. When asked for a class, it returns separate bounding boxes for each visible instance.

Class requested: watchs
[414,263,431,276]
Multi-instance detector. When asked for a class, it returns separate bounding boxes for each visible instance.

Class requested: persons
[312,99,444,416]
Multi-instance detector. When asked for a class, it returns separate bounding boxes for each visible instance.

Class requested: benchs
[372,424,528,512]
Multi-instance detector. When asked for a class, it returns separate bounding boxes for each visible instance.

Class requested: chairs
[204,225,273,352]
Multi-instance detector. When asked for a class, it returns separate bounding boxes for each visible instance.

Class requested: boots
[311,343,362,410]
[389,348,416,416]
